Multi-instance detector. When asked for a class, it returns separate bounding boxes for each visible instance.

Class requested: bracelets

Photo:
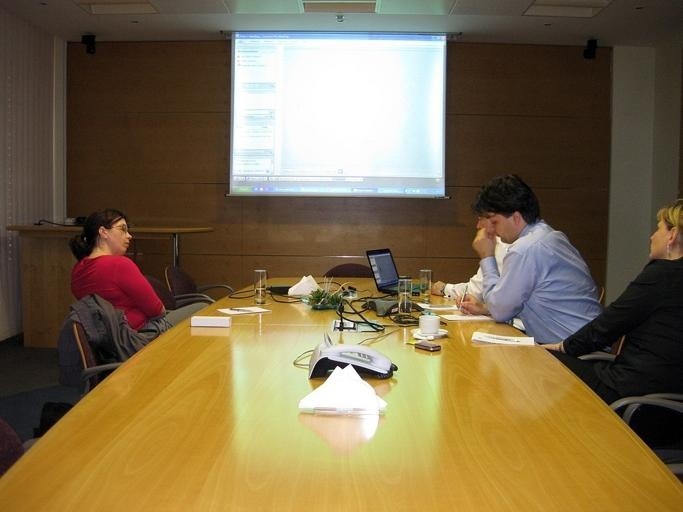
[558,340,564,353]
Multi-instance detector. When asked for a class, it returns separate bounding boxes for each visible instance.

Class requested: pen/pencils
[313,407,363,411]
[230,307,252,312]
[459,286,468,311]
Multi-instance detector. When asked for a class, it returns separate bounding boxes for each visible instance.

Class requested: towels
[299,363,389,415]
[288,275,323,297]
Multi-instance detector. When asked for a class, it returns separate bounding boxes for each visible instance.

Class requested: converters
[271,287,292,293]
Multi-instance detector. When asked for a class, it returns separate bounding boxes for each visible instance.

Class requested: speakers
[86,35,96,54]
[586,39,597,59]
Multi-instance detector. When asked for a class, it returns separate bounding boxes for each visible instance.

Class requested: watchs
[440,282,446,296]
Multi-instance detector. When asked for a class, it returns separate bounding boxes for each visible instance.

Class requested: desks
[0,277,683,511]
[127,225,215,267]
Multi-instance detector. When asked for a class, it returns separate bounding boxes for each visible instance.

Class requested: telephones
[309,342,398,380]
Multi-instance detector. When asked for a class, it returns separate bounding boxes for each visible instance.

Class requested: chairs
[164,263,235,310]
[323,263,374,278]
[609,396,682,483]
[597,284,605,303]
[575,334,683,464]
[0,418,25,479]
[72,293,123,394]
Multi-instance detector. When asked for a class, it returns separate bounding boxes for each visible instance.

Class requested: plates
[410,327,449,339]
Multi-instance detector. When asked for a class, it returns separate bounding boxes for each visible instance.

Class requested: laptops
[367,249,433,296]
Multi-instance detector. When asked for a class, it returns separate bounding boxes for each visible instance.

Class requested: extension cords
[339,290,358,300]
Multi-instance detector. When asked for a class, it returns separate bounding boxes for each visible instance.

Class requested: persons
[68,208,209,341]
[430,187,510,302]
[454,174,613,357]
[539,200,683,450]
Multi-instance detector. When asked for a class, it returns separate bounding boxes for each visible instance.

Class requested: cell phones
[415,341,441,351]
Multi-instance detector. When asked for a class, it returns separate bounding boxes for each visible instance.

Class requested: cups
[252,268,268,305]
[418,269,433,303]
[396,275,414,315]
[416,313,441,335]
